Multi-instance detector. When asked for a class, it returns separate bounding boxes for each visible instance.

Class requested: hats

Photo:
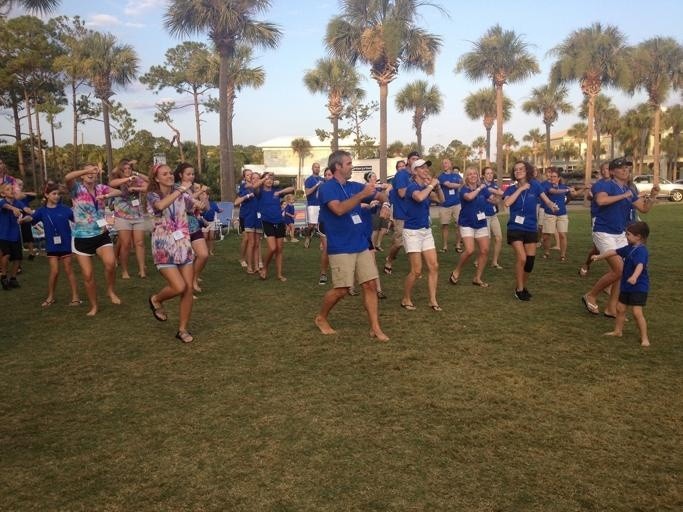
[609,157,633,169]
[411,158,432,172]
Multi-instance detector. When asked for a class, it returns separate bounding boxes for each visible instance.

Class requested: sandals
[377,290,387,299]
[239,258,287,283]
[347,287,361,296]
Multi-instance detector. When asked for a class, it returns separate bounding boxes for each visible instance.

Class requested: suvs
[632,174,682,201]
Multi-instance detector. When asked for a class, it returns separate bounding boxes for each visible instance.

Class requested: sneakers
[1,266,22,291]
[284,235,300,244]
[318,271,328,285]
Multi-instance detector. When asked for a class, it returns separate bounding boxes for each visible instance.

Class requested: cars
[560,177,601,204]
[499,177,515,198]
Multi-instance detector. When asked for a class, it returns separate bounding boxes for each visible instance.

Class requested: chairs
[213,200,240,243]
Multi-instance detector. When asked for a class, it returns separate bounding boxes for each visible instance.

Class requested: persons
[1,161,224,343]
[234,168,300,283]
[577,155,661,346]
[304,150,587,346]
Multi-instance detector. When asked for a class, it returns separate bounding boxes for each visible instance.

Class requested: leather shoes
[107,292,121,305]
[137,271,146,279]
[121,275,130,279]
[86,311,95,316]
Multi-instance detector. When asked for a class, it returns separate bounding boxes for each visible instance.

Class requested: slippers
[41,299,56,307]
[401,302,417,311]
[375,242,394,276]
[472,281,489,288]
[603,309,630,322]
[69,299,83,307]
[578,263,588,279]
[448,272,461,284]
[440,239,567,270]
[149,294,168,322]
[427,303,443,311]
[581,295,600,315]
[175,328,194,343]
